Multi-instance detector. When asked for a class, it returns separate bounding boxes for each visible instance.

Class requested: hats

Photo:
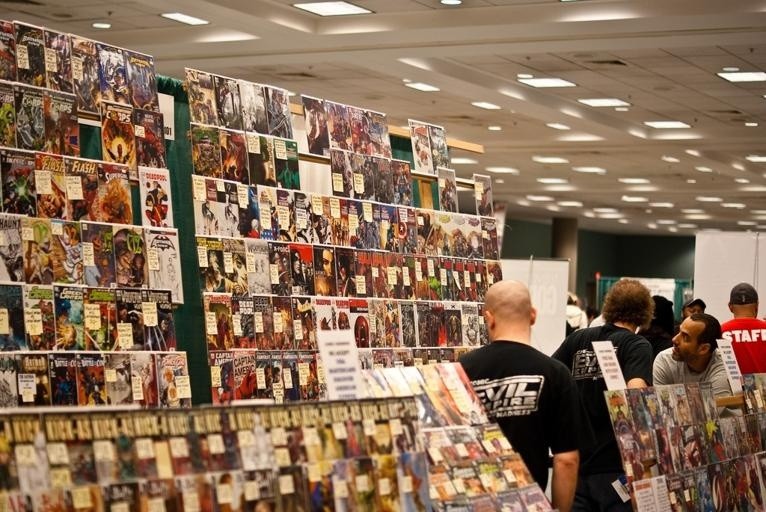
[683,297,706,308]
[730,283,758,304]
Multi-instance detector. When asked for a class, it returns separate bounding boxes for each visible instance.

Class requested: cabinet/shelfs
[0,14,763,512]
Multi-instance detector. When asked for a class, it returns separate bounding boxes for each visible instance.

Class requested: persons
[551,278,766,511]
[626,386,758,479]
[461,277,581,512]
